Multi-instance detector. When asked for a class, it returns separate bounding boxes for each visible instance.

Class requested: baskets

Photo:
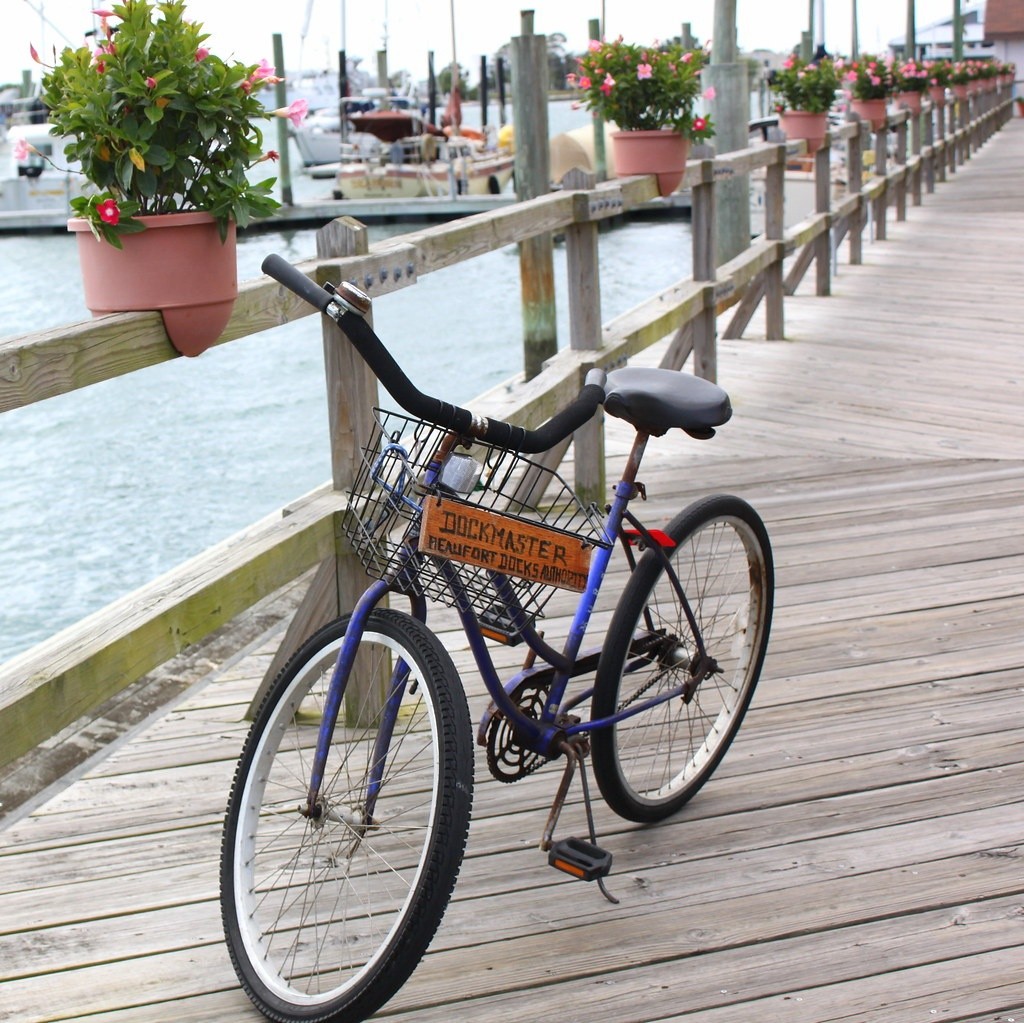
[343,408,615,637]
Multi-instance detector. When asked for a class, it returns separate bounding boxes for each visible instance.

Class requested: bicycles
[216,253,779,1023]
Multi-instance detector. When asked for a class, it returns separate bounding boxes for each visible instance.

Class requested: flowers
[767,44,1015,113]
[14,0,311,248]
[562,34,718,148]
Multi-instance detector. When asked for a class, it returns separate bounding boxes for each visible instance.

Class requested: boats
[0,0,517,229]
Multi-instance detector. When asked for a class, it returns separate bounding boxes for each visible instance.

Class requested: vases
[776,75,1017,153]
[610,127,688,197]
[67,207,237,356]
